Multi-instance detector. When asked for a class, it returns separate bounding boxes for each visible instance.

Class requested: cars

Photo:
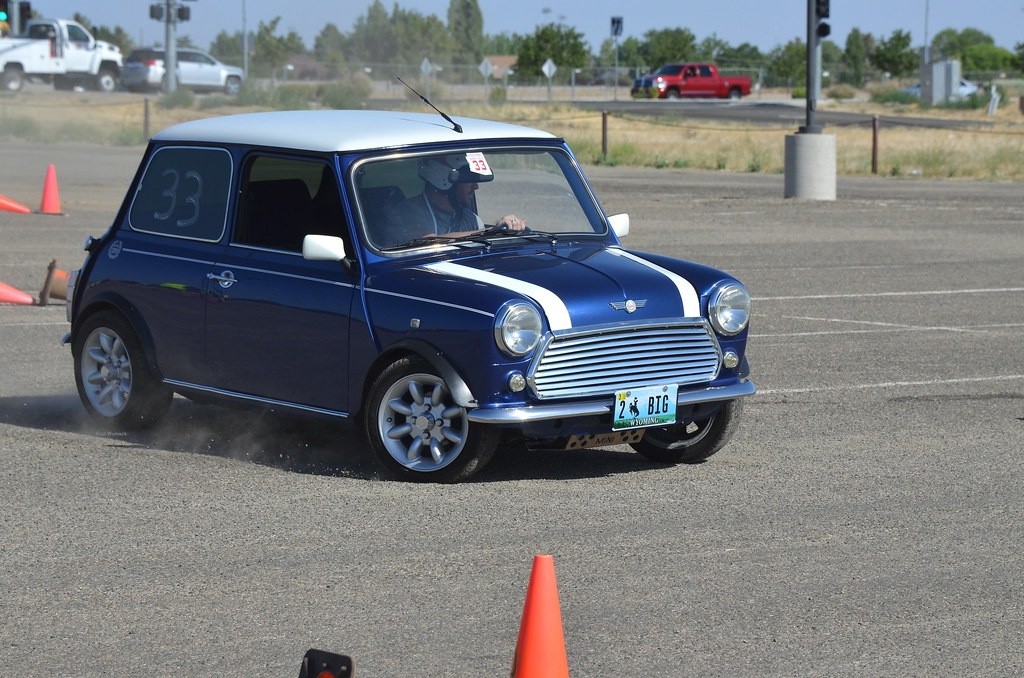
[903,78,977,104]
[123,47,247,97]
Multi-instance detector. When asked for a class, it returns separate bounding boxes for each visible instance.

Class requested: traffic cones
[0,194,31,213]
[35,162,62,214]
[0,282,37,306]
[510,556,571,678]
[301,648,354,678]
[43,260,71,305]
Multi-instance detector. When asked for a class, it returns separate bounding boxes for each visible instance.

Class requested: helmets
[418,152,494,191]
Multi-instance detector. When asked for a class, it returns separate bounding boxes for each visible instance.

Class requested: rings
[511,219,516,222]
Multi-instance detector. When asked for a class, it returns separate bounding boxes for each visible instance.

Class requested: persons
[387,154,527,244]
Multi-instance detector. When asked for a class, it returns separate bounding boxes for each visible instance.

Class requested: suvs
[61,110,754,481]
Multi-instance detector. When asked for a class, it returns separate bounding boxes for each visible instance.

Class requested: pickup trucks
[0,20,123,92]
[631,63,751,102]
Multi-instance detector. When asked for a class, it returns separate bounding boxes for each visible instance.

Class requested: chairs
[243,179,314,255]
[359,185,407,248]
[315,163,367,255]
